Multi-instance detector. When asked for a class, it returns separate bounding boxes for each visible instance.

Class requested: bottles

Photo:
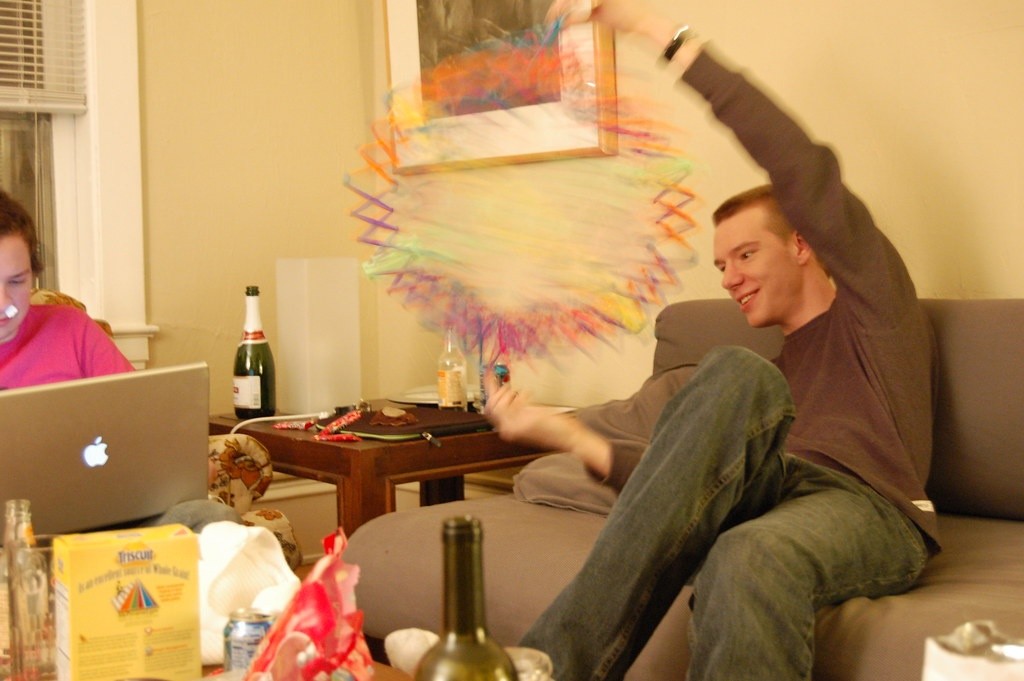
[415,516,518,681]
[438,322,468,413]
[0,498,48,681]
[233,286,276,419]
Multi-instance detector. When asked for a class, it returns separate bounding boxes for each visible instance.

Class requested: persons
[0,189,137,392]
[485,1,944,681]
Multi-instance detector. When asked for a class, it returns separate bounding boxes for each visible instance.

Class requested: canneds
[478,363,510,405]
[223,609,276,674]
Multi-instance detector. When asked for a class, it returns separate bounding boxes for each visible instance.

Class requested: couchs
[343,298,1024,681]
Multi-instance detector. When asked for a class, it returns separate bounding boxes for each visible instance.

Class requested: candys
[273,409,364,443]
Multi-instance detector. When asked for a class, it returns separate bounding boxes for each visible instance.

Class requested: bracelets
[658,22,692,61]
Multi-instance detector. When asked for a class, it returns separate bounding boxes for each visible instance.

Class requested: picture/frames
[383,0,618,175]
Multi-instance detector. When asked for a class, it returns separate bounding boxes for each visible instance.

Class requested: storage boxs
[53,523,202,681]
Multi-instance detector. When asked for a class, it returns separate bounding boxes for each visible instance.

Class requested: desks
[209,406,563,540]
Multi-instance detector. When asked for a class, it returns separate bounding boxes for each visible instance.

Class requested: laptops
[0,361,209,539]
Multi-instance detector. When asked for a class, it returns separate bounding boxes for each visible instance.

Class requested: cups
[7,535,54,680]
